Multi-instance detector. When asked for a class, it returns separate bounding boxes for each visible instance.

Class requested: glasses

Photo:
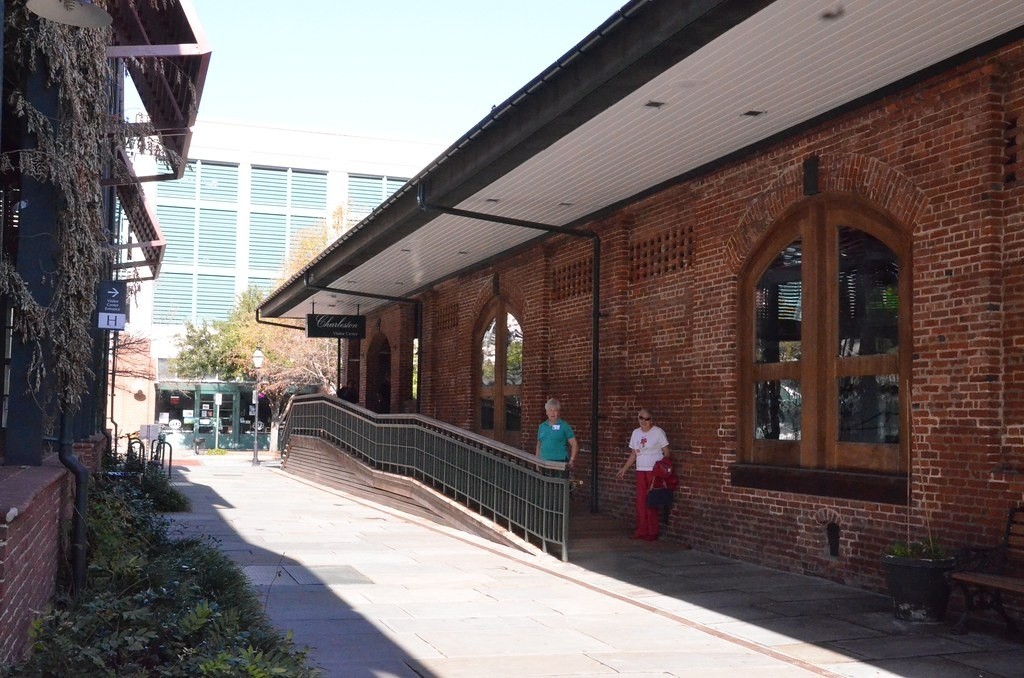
[639,416,651,421]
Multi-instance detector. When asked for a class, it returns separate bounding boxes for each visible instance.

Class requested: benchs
[943,507,1024,641]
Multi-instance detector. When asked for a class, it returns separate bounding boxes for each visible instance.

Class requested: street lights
[252,347,264,466]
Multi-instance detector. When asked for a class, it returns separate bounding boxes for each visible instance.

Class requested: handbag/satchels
[646,476,673,507]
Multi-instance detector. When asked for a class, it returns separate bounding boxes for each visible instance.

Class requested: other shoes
[645,535,657,541]
[629,534,645,539]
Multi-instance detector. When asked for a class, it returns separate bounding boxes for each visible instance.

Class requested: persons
[336,379,357,405]
[535,397,579,519]
[616,409,670,543]
[258,389,271,422]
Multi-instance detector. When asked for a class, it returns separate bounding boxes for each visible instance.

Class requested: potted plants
[881,539,955,626]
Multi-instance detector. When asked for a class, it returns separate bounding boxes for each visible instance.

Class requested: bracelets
[570,457,574,461]
